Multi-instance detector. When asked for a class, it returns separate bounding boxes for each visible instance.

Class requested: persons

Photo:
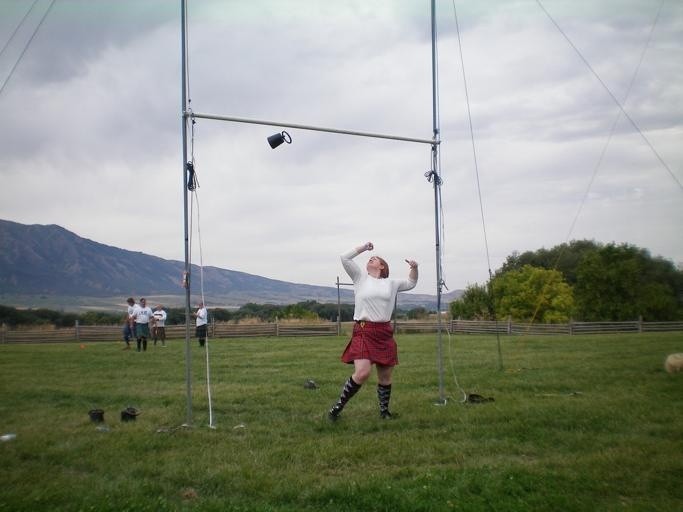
[193,303,208,347]
[150,303,167,347]
[122,297,137,350]
[130,298,154,352]
[327,242,419,420]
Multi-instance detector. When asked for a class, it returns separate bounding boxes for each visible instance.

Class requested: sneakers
[328,405,340,421]
[381,410,394,421]
